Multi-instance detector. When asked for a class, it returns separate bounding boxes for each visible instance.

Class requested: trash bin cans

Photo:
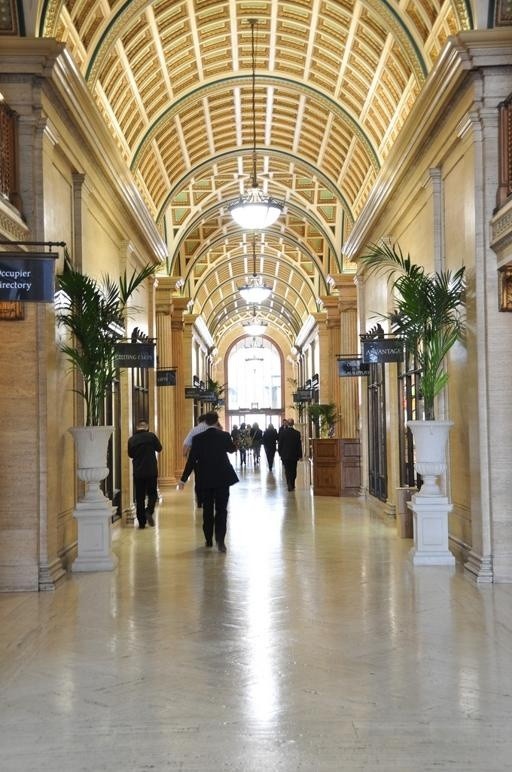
[396,488,420,538]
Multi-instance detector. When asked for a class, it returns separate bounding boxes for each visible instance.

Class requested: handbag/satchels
[245,437,253,448]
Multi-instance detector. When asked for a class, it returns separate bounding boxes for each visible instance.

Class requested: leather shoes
[138,511,155,530]
[204,538,227,552]
[197,502,202,508]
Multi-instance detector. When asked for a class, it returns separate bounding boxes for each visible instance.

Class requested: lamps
[224,18,285,306]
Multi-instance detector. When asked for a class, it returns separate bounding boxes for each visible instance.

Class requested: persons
[178,411,239,552]
[128,420,162,529]
[231,419,302,491]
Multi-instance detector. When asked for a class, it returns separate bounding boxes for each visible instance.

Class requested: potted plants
[49,244,167,574]
[360,238,466,569]
